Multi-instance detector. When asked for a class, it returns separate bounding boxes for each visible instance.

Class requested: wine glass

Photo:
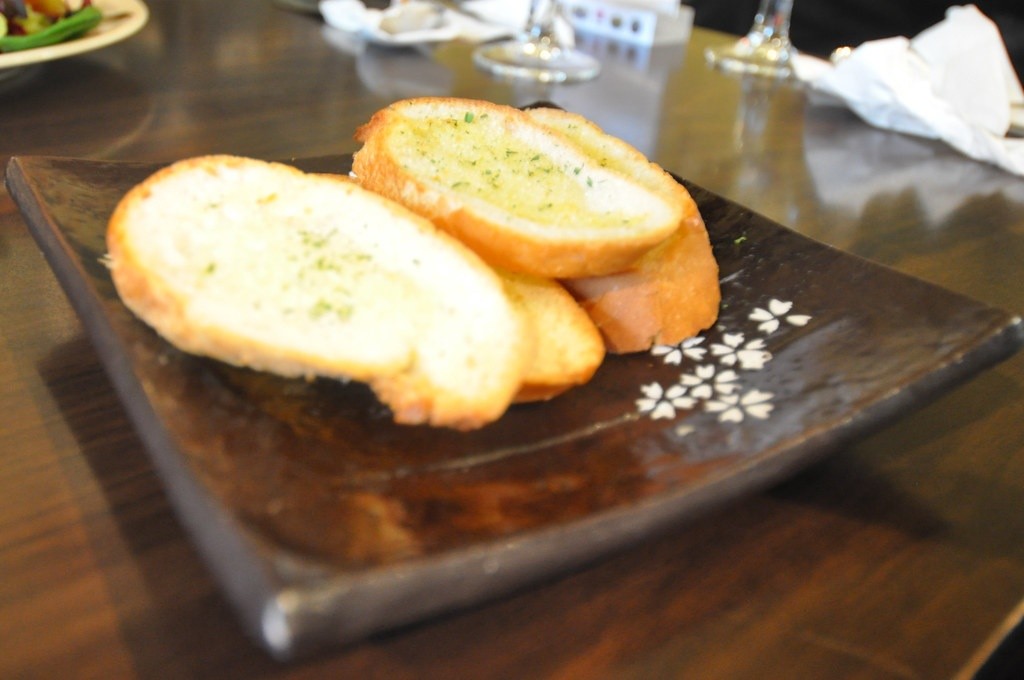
[474,0,602,83]
[707,0,803,77]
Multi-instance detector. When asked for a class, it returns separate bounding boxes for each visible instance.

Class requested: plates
[0,0,150,70]
[7,99,1023,664]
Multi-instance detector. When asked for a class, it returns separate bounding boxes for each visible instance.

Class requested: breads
[107,98,722,432]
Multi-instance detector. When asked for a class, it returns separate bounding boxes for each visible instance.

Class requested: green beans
[0,6,102,51]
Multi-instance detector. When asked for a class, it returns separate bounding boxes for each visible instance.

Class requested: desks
[0,0,1024,680]
